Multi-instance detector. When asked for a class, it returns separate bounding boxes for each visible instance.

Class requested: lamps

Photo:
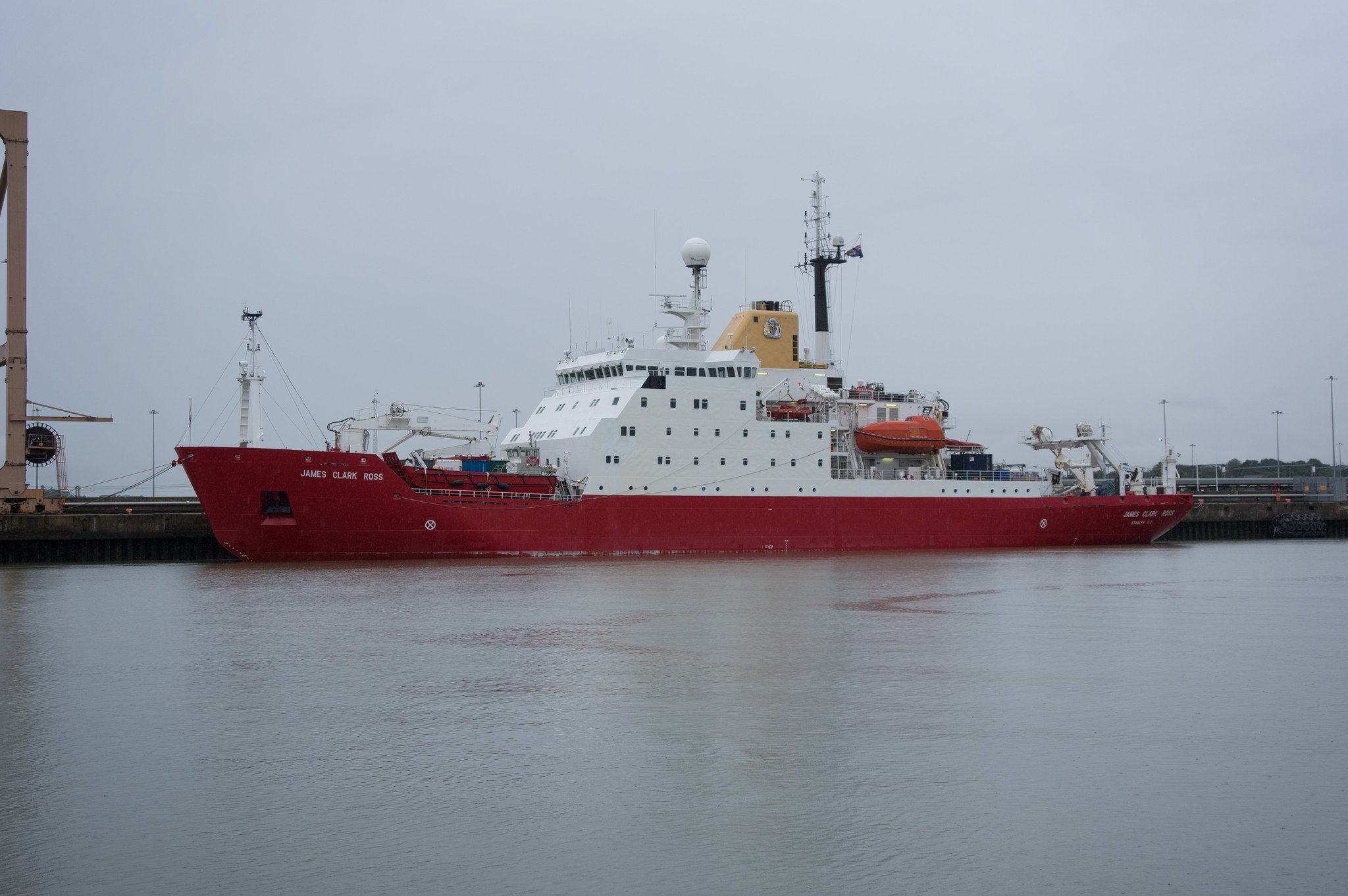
[606,349,623,355]
[239,361,248,372]
[558,355,578,364]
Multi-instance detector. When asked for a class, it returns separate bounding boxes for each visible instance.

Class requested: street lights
[1336,443,1344,478]
[1272,410,1283,478]
[1323,376,1340,478]
[511,409,522,428]
[147,409,159,498]
[1189,444,1196,479]
[1160,400,1170,457]
[473,382,486,439]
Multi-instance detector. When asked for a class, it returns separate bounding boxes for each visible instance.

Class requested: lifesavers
[526,456,540,466]
[1277,512,1327,539]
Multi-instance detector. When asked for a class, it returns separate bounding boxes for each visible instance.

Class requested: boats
[944,437,984,452]
[854,414,949,455]
[165,171,1202,561]
[766,403,813,420]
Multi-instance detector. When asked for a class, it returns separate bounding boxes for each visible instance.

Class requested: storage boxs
[463,460,509,472]
